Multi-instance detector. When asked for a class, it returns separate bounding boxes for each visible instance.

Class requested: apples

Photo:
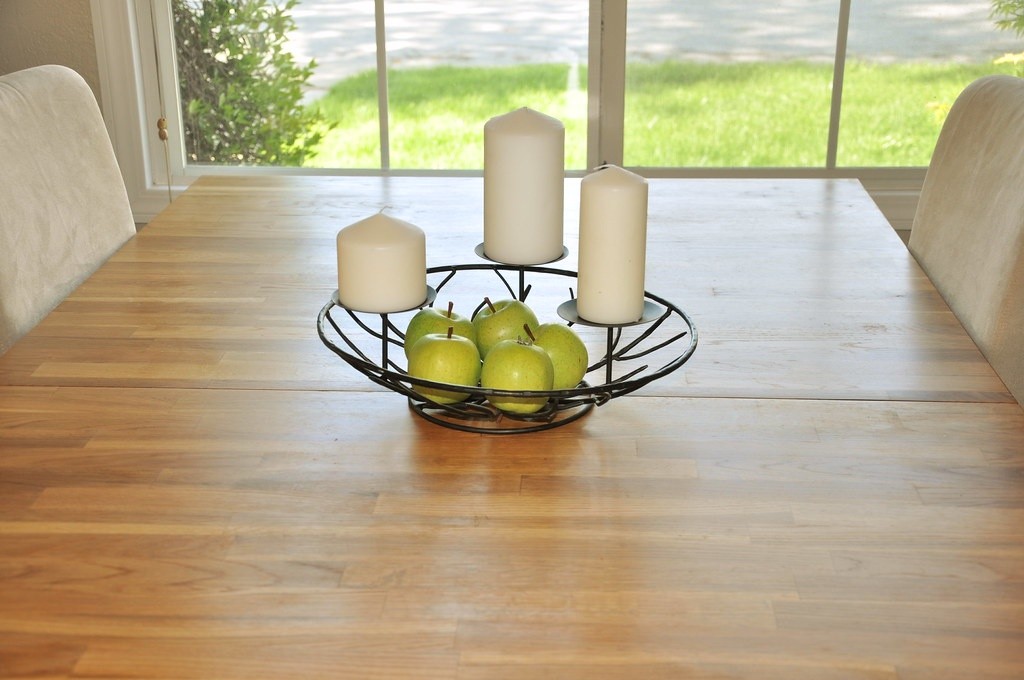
[472,297,539,360]
[407,325,481,404]
[480,336,554,413]
[404,300,477,360]
[523,322,588,390]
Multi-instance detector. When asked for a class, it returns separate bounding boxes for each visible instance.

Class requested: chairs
[907,75,1024,409]
[0,64,136,357]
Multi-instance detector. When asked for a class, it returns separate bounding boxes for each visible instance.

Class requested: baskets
[317,261,698,433]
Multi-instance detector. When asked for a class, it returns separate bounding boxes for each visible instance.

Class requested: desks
[0,176,1024,680]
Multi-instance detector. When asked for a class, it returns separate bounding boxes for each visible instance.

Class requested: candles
[337,205,426,313]
[483,108,563,265]
[576,164,649,323]
